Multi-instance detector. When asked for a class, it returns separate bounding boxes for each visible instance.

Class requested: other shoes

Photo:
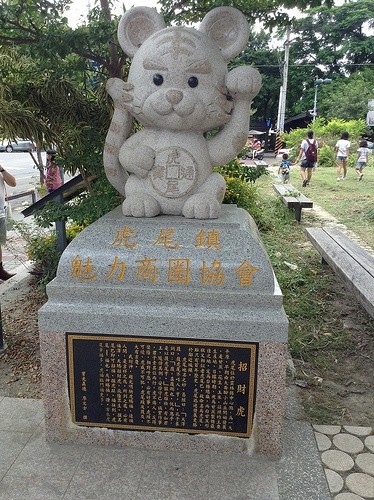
[359,174,363,181]
[303,179,308,187]
[0,270,16,281]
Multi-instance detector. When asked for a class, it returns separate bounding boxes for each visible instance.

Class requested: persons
[278,154,295,184]
[295,130,318,187]
[45,150,62,193]
[252,139,261,160]
[0,165,16,280]
[355,141,369,179]
[335,132,351,181]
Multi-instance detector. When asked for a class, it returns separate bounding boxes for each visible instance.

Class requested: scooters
[242,144,266,160]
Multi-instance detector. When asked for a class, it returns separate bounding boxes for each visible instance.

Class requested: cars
[275,146,294,163]
[0,135,36,153]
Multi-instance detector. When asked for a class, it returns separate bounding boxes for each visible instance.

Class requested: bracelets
[1,168,5,172]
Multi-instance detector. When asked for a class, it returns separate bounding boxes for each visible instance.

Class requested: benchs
[254,160,268,168]
[306,226,374,318]
[274,183,313,221]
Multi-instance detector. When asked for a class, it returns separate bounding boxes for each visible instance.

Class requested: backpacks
[305,139,317,163]
[281,162,289,175]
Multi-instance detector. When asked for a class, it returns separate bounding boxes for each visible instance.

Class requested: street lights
[313,74,332,122]
